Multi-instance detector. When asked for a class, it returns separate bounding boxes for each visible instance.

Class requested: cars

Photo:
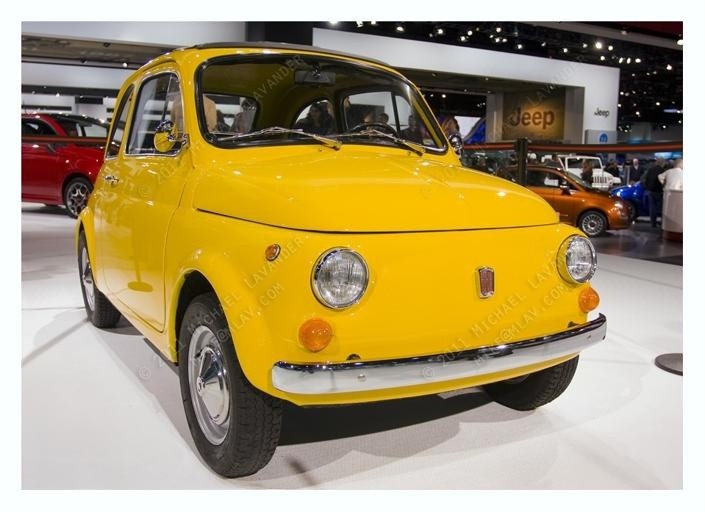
[70,39,609,481]
[467,153,663,237]
[21,109,125,220]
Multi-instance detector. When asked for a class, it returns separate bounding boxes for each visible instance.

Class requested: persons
[461,152,683,228]
[169,89,425,145]
